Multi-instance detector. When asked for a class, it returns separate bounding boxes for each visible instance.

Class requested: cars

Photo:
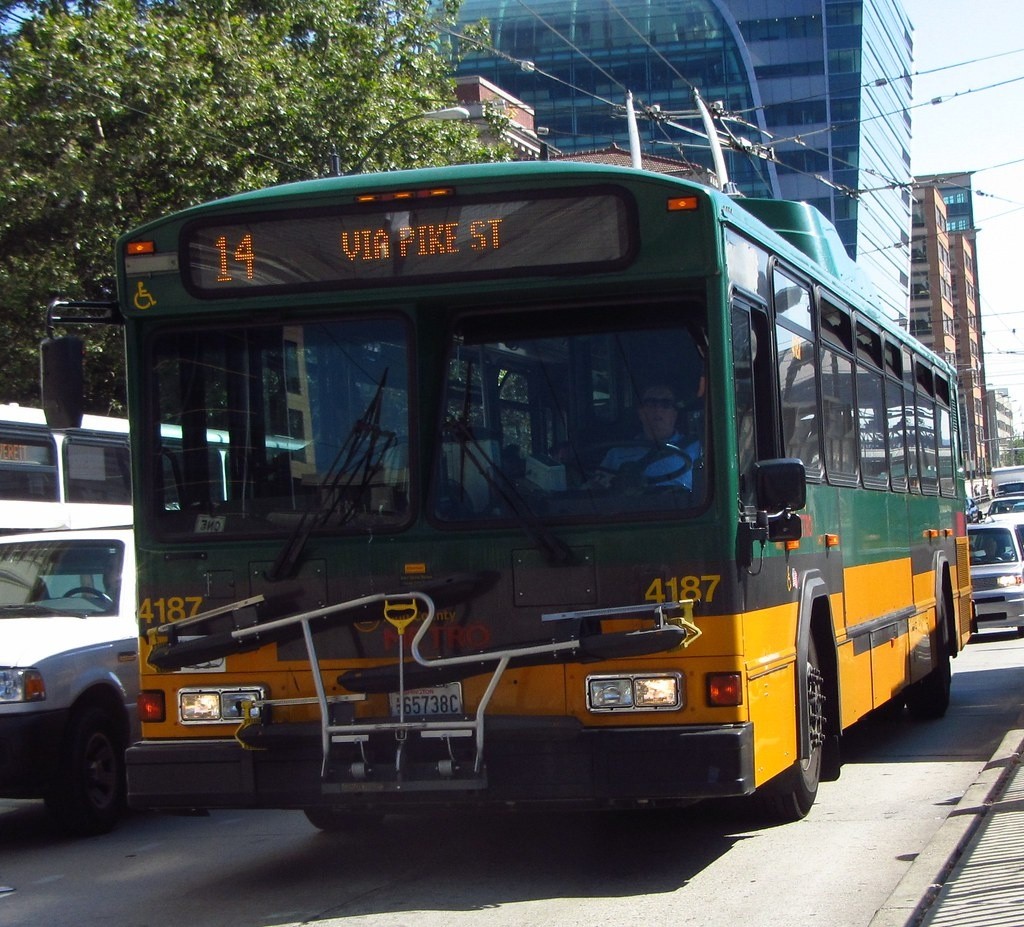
[963,497,983,525]
[967,524,1024,636]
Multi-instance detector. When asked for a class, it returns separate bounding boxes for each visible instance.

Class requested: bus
[0,405,309,607]
[40,94,976,842]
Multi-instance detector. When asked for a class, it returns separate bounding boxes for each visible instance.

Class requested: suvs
[977,466,1024,527]
[0,530,139,837]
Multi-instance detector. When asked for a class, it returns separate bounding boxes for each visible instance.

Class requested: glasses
[642,397,675,409]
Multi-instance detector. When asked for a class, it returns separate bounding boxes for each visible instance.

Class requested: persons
[983,537,1010,563]
[578,378,701,494]
[537,439,579,478]
[94,554,122,613]
